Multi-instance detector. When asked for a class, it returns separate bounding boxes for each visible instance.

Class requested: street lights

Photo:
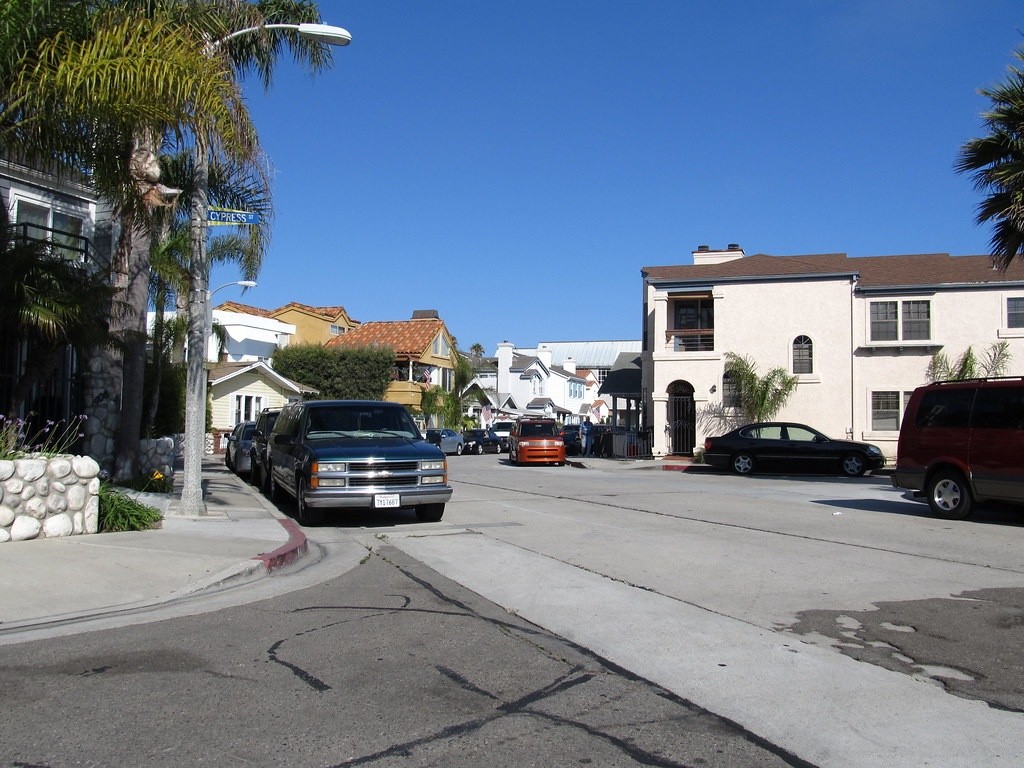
[183,23,354,518]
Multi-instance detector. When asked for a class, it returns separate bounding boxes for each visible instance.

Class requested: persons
[483,430,490,439]
[579,416,595,458]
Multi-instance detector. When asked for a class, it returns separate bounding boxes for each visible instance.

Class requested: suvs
[890,374,1024,521]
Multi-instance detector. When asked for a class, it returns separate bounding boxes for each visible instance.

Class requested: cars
[229,421,256,479]
[702,421,885,479]
[489,417,606,454]
[508,418,566,467]
[223,423,240,467]
[248,405,289,498]
[461,429,502,454]
[264,399,455,522]
[422,427,464,456]
